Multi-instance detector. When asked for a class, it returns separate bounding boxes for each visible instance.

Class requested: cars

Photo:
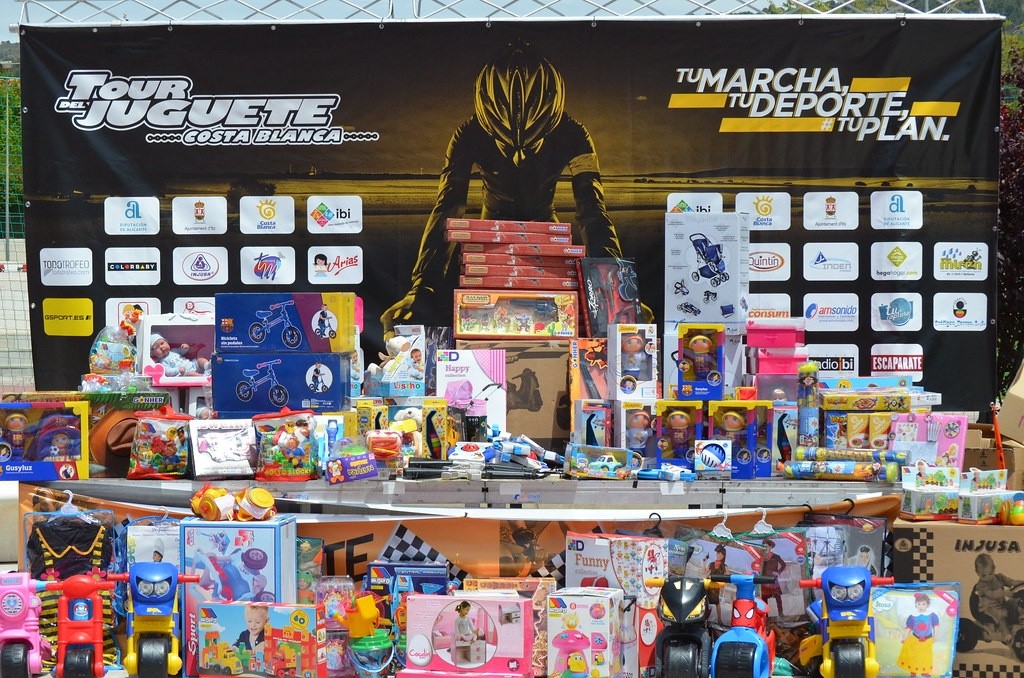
[587,452,623,472]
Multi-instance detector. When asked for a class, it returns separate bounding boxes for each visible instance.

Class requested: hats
[762,537,775,548]
[154,539,164,557]
[87,406,139,477]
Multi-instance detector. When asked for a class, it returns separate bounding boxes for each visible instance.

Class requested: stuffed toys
[368,335,409,376]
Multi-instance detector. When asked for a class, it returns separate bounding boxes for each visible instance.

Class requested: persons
[663,409,695,460]
[627,409,655,458]
[761,537,786,617]
[150,333,208,377]
[682,334,717,381]
[312,362,321,390]
[232,605,269,657]
[411,349,423,372]
[975,553,1024,642]
[715,409,747,458]
[622,333,651,381]
[52,433,72,455]
[2,408,37,460]
[703,544,728,629]
[455,601,475,635]
[769,386,788,402]
[318,304,328,338]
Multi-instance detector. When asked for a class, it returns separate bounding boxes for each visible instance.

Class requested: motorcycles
[796,564,895,678]
[105,561,201,678]
[709,572,777,678]
[0,570,58,678]
[645,576,726,678]
[46,574,115,678]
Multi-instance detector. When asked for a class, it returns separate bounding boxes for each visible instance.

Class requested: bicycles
[308,373,329,393]
[248,299,303,349]
[315,316,336,339]
[235,359,289,407]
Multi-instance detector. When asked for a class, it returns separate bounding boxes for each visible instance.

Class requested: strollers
[689,233,730,287]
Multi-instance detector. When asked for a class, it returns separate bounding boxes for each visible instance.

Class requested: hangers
[841,499,853,515]
[748,506,775,534]
[156,506,169,526]
[641,512,664,538]
[708,510,732,539]
[795,504,818,528]
[44,489,91,525]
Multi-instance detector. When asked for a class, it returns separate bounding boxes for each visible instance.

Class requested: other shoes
[1001,633,1013,642]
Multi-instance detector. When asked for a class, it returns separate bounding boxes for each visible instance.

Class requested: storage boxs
[0,212,1023,678]
